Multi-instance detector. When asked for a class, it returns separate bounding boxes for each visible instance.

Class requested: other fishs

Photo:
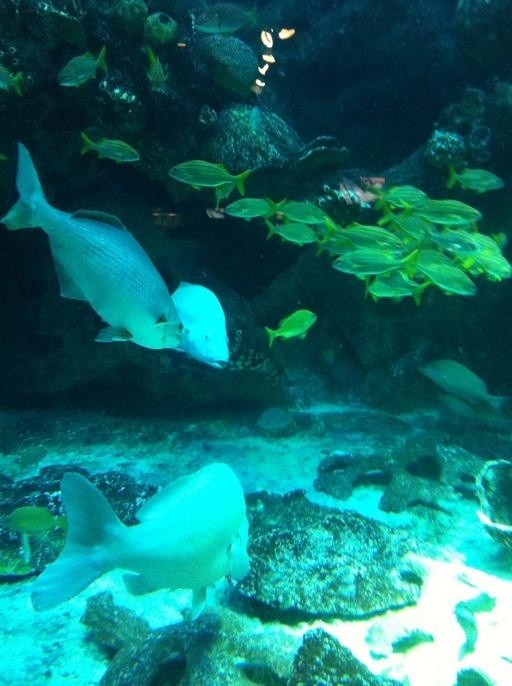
[219,161,512,306]
